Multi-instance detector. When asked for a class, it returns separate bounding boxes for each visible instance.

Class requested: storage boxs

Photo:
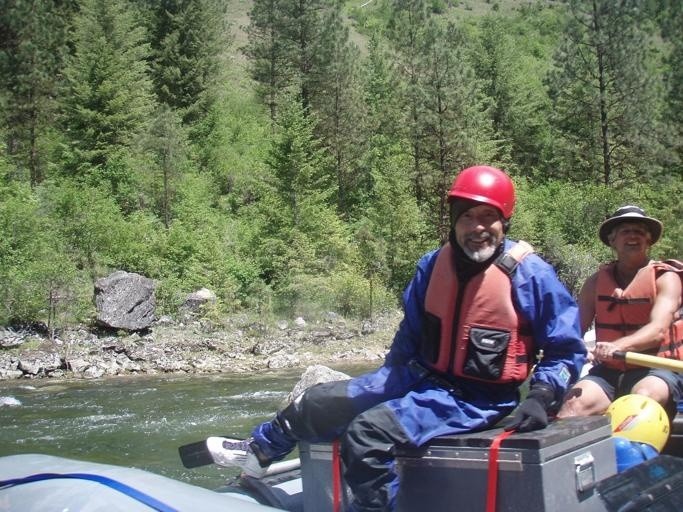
[296,410,619,511]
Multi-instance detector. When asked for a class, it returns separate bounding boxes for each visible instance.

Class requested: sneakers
[207,437,268,479]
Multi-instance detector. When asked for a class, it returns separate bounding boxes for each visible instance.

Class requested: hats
[599,206,663,246]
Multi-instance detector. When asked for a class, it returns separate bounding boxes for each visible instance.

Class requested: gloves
[505,385,558,433]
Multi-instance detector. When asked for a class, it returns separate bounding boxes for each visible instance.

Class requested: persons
[203,165,588,512]
[554,205,681,419]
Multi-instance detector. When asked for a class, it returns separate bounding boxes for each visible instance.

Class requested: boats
[0,371,682,510]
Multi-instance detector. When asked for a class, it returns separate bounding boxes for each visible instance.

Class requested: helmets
[448,165,517,219]
[605,394,670,452]
